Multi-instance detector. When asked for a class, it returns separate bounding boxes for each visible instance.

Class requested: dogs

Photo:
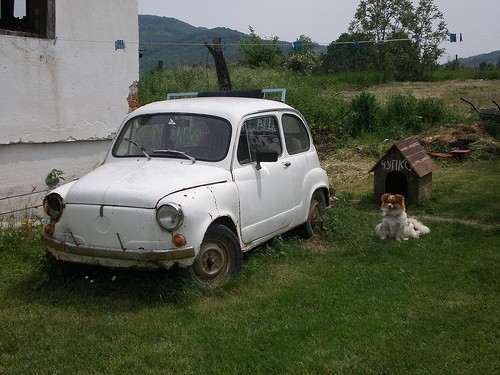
[374,193,431,243]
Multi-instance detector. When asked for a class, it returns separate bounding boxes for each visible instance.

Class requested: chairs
[284,135,302,155]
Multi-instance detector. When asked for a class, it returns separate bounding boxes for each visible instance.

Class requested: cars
[43,95,332,292]
